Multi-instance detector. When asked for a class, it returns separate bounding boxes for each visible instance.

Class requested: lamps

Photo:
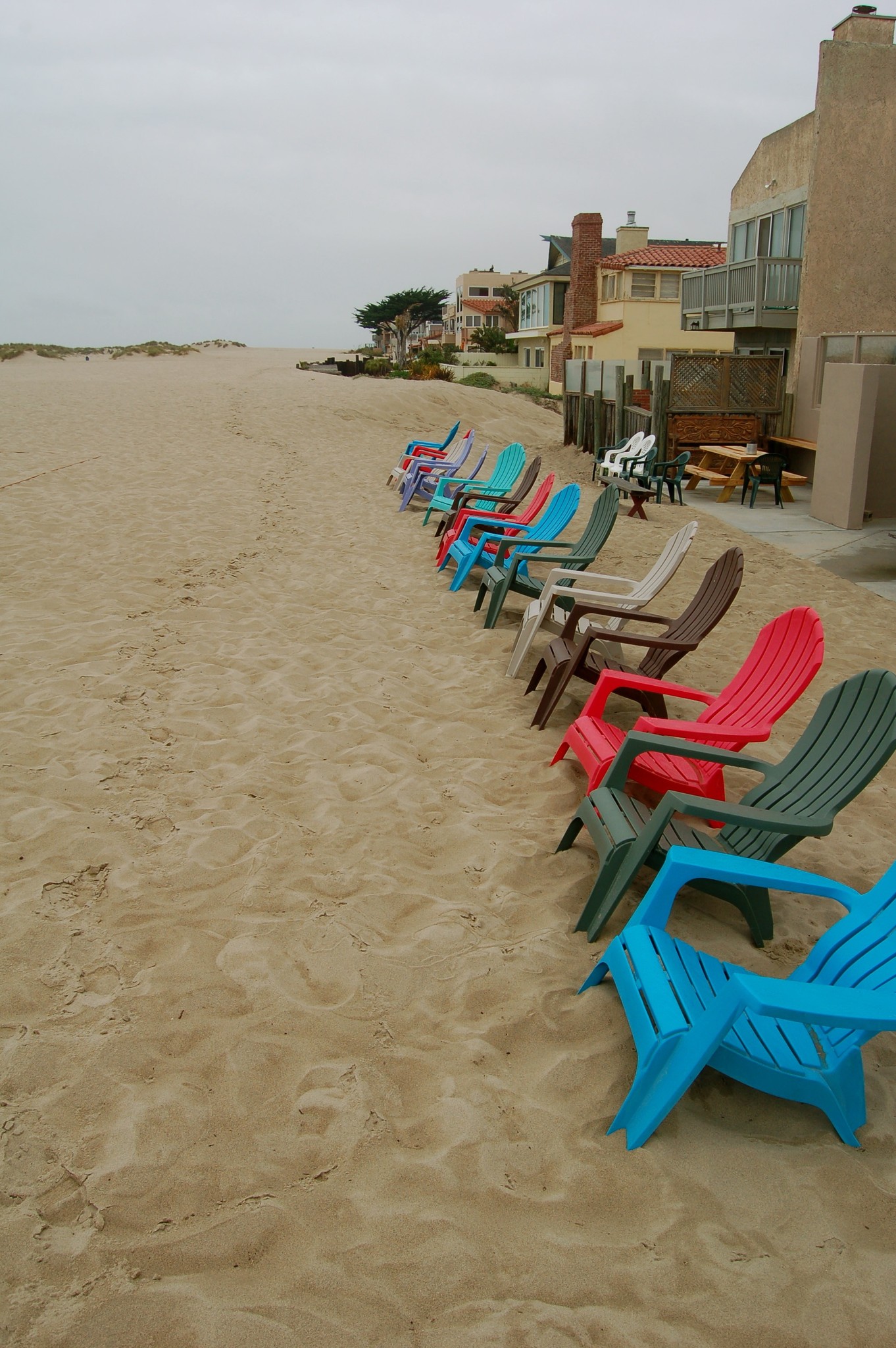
[690,320,700,331]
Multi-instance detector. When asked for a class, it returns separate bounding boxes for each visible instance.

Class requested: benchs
[597,474,657,521]
[676,462,730,481]
[748,464,808,481]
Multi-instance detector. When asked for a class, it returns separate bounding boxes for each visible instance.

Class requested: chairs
[387,417,896,1151]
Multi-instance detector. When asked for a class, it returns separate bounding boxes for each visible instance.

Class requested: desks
[684,445,807,503]
[770,436,817,450]
